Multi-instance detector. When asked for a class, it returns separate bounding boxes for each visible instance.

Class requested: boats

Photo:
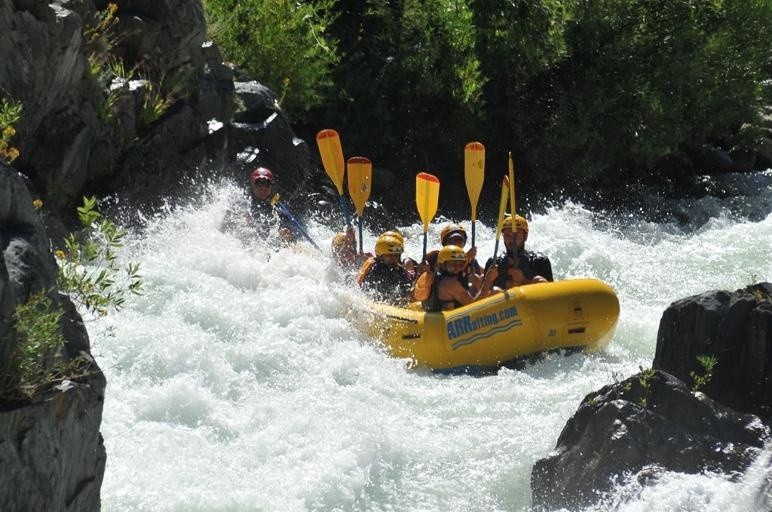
[285,244,619,373]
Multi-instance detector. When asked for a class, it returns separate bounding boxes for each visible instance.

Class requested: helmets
[250,167,272,184]
[375,231,403,256]
[441,224,467,244]
[332,234,346,248]
[501,215,528,231]
[437,245,466,266]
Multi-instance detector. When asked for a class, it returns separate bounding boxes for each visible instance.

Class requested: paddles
[508,151,518,268]
[415,172,440,273]
[464,142,485,289]
[490,176,511,295]
[317,129,355,244]
[347,156,372,268]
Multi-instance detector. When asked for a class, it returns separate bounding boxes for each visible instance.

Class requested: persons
[217,167,299,259]
[324,215,553,313]
